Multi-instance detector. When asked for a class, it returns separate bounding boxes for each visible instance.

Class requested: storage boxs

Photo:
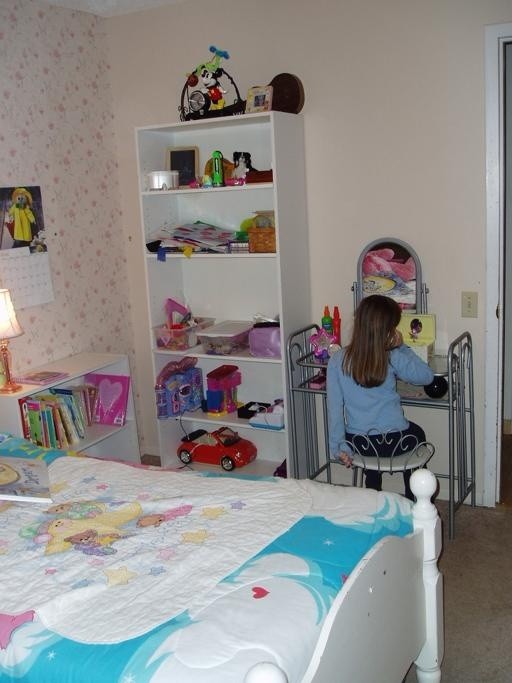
[396,314,436,365]
[152,315,215,352]
[196,319,255,355]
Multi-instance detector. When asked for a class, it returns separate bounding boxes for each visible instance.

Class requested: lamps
[1,290,23,393]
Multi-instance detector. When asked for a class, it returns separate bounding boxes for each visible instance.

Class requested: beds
[0,450,442,683]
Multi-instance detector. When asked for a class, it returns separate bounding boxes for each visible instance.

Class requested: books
[13,370,70,385]
[1,456,51,503]
[84,371,129,426]
[19,384,102,454]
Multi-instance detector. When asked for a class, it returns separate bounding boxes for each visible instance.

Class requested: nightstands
[1,354,141,466]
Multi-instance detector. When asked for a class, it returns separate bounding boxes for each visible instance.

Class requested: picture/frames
[244,86,272,114]
[165,146,200,188]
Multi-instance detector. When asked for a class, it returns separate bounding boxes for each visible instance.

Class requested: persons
[325,296,434,498]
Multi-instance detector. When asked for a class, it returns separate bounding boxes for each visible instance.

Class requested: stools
[341,427,434,500]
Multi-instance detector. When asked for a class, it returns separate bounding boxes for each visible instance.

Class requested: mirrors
[353,238,427,316]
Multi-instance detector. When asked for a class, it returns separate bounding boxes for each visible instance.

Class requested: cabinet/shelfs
[133,112,320,482]
